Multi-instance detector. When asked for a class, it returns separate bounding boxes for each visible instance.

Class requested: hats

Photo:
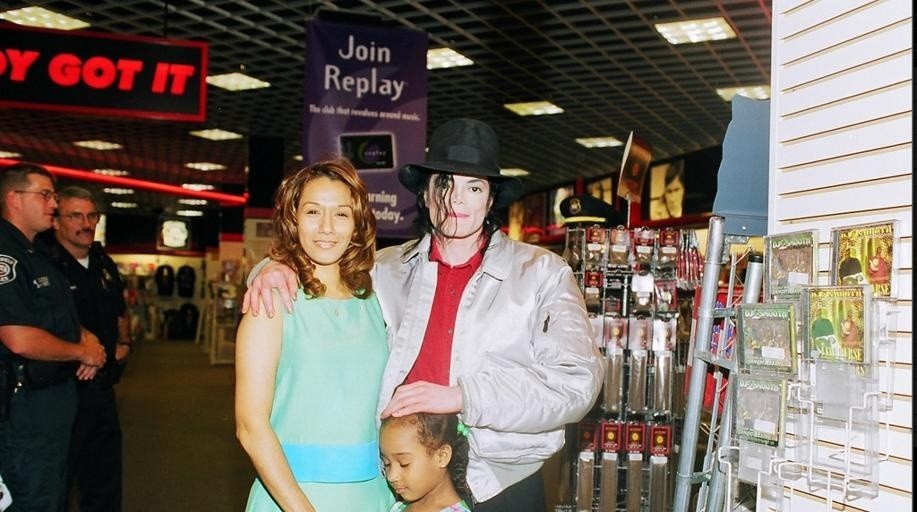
[557,194,617,231]
[398,116,525,212]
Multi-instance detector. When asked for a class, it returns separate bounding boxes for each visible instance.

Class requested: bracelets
[118,341,132,350]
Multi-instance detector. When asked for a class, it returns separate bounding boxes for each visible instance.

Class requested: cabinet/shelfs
[194,252,250,365]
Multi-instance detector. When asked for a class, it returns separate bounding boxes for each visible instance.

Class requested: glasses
[15,188,61,202]
[59,211,100,225]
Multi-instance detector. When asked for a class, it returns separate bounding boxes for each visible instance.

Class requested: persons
[33,184,134,512]
[809,310,835,347]
[379,413,475,512]
[839,248,863,283]
[241,116,606,512]
[234,156,399,512]
[1,161,108,511]
[839,310,859,346]
[869,246,889,285]
[653,160,687,220]
